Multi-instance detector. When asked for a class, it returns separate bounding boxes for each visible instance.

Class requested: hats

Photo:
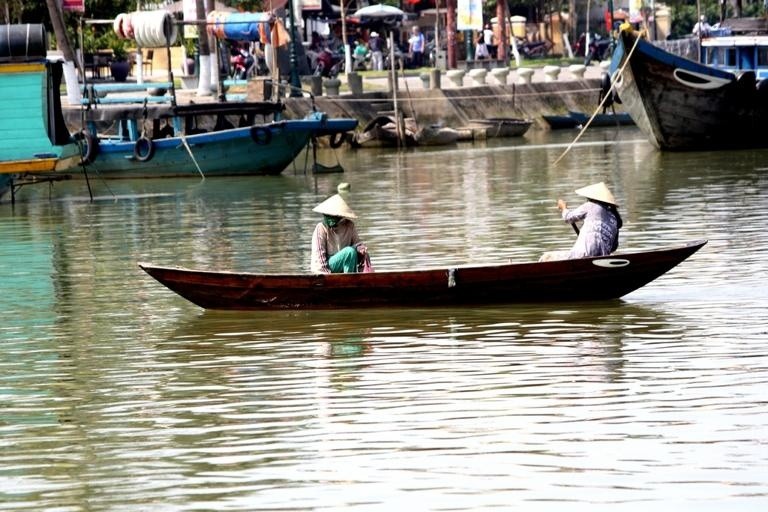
[311,193,359,222]
[572,182,620,208]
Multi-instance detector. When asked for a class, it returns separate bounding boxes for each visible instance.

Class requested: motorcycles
[510,37,548,59]
[572,32,612,68]
[231,38,475,79]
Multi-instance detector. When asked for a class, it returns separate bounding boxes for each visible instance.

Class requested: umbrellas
[354,2,404,25]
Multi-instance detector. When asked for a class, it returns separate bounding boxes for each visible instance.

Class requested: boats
[66,111,360,180]
[608,15,768,155]
[137,238,710,312]
[357,111,635,148]
[152,308,706,343]
[0,23,84,207]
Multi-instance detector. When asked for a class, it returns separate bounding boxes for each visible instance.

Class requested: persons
[537,181,623,263]
[248,23,495,73]
[310,193,370,274]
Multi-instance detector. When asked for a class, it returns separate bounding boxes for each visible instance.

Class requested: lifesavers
[112,10,178,48]
